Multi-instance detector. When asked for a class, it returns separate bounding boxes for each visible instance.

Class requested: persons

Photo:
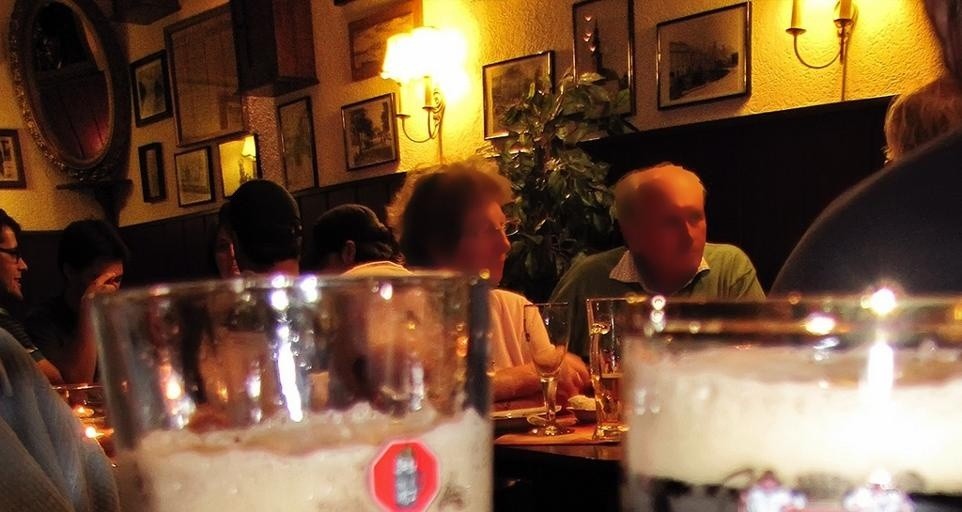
[751,0,962,512]
[541,160,767,364]
[0,208,126,411]
[302,200,402,398]
[118,177,325,403]
[0,327,124,512]
[325,158,592,430]
[206,201,243,284]
[32,217,143,392]
[881,65,962,166]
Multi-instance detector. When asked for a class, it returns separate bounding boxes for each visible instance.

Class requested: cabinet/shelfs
[232,2,321,98]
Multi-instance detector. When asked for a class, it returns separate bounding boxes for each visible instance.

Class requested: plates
[491,403,562,423]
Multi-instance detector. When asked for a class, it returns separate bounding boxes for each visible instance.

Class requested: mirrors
[6,1,132,192]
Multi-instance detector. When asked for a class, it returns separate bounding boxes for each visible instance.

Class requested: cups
[615,283,961,512]
[585,295,627,444]
[91,261,497,512]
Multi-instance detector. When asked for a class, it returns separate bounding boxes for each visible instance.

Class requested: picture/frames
[160,2,248,147]
[655,0,752,111]
[345,0,421,82]
[137,139,168,205]
[275,90,319,195]
[481,49,556,141]
[571,0,637,119]
[339,91,401,172]
[220,132,261,200]
[128,48,173,127]
[173,143,217,208]
[0,129,26,190]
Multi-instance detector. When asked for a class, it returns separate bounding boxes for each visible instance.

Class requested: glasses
[2,246,20,263]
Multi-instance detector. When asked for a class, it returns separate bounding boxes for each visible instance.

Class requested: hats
[219,179,304,245]
[314,204,387,257]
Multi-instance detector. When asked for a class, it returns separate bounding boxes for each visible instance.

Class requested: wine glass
[519,300,574,439]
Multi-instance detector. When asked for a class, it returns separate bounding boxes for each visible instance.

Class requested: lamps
[783,1,860,71]
[379,27,465,144]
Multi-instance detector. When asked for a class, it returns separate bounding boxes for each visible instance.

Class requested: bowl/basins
[566,405,601,423]
